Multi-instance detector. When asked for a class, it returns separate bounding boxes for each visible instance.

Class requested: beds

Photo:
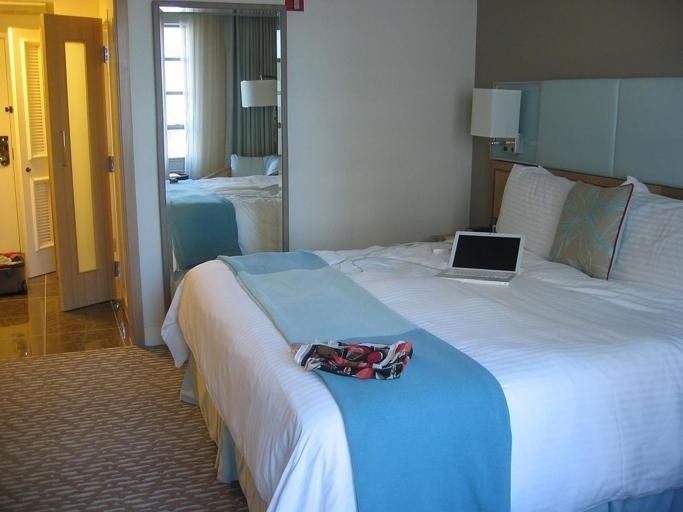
[176,158,681,511]
[165,176,283,275]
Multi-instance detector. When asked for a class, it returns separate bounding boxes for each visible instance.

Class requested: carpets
[0,345,248,512]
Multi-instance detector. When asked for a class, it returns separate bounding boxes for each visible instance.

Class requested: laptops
[432,230,525,287]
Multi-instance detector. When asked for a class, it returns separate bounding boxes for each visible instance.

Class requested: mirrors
[151,0,289,317]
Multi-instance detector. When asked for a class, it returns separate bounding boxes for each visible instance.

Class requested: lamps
[468,87,524,155]
[240,72,278,107]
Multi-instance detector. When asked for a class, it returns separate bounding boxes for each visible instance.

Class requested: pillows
[494,162,682,293]
[230,154,281,177]
